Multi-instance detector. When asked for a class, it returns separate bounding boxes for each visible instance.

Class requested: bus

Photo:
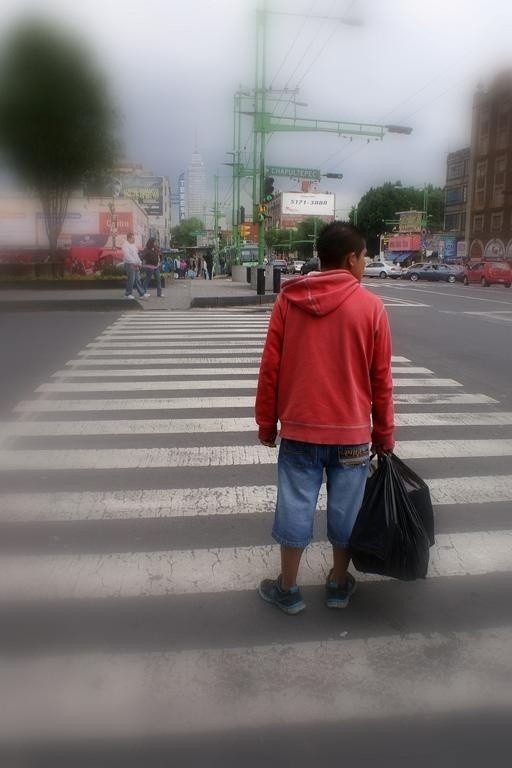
[219,241,264,275]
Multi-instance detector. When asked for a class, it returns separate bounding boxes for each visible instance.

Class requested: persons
[120,233,150,299]
[69,252,225,280]
[142,238,166,297]
[253,219,396,616]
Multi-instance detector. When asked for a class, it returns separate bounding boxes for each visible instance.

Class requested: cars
[401,263,465,282]
[271,258,320,275]
[364,261,402,279]
[463,261,512,288]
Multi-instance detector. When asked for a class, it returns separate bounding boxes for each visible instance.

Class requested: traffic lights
[264,178,275,203]
[389,125,413,134]
[327,173,343,179]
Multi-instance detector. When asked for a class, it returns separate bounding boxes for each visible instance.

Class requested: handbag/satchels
[337,444,441,583]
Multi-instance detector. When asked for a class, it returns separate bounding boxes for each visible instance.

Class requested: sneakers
[259,574,307,615]
[325,568,357,609]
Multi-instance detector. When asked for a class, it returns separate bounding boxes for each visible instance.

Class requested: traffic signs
[267,166,320,179]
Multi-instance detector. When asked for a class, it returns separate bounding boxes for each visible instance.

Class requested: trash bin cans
[246,266,251,283]
[273,268,280,293]
[257,268,265,295]
